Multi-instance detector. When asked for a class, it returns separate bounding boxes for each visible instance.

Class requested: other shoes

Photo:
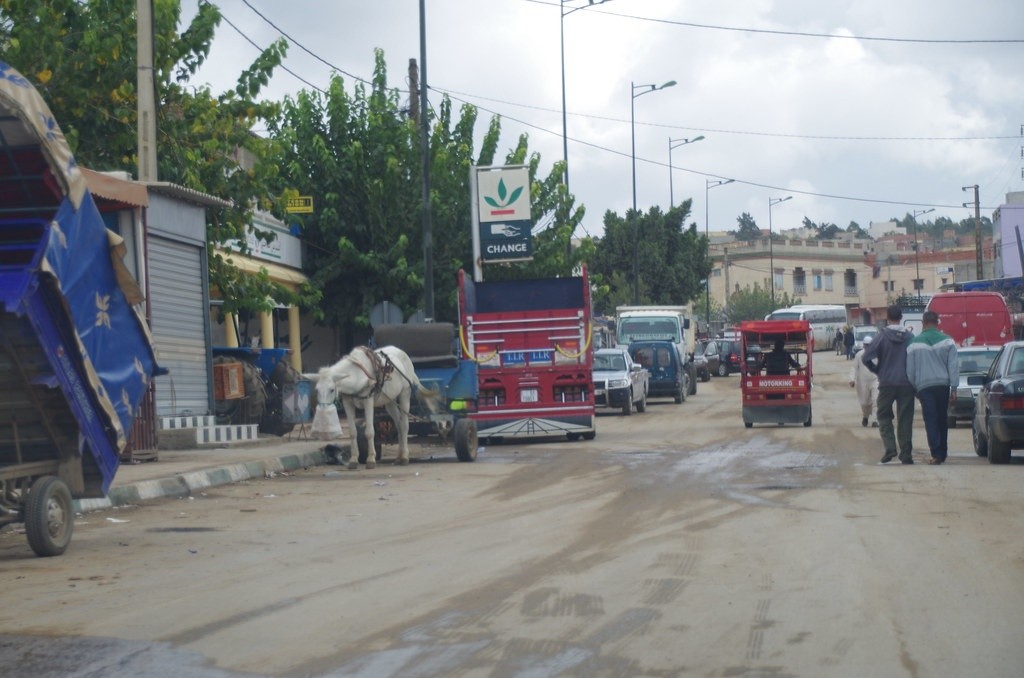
[930,457,940,465]
[881,449,897,463]
[862,417,868,426]
[872,422,878,427]
[899,453,913,464]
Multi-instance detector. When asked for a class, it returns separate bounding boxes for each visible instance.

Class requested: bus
[764,304,848,352]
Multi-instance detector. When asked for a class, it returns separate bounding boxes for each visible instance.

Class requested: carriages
[299,321,482,469]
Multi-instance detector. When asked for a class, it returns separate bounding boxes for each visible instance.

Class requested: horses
[300,344,433,471]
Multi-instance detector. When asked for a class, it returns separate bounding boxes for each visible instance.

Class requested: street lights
[704,178,736,337]
[912,207,937,305]
[669,135,706,212]
[768,195,793,311]
[629,80,678,306]
[559,0,614,257]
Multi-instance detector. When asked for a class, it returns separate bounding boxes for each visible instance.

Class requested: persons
[849,337,878,426]
[762,341,801,375]
[836,328,843,355]
[862,306,916,464]
[906,312,959,464]
[844,329,854,361]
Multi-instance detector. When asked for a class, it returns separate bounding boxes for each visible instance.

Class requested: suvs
[626,340,691,405]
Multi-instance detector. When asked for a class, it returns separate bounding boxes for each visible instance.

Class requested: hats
[863,337,872,344]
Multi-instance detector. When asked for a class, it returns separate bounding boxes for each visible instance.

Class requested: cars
[688,325,743,382]
[589,348,650,418]
[966,341,1024,465]
[945,346,1004,428]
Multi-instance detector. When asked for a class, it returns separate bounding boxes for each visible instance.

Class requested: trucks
[616,303,699,396]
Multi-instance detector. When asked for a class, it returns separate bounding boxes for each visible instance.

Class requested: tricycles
[0,62,171,560]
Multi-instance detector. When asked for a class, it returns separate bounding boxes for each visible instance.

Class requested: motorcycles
[740,320,815,428]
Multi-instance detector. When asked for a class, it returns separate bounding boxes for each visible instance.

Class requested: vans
[898,312,924,336]
[921,291,1015,349]
[853,326,880,354]
